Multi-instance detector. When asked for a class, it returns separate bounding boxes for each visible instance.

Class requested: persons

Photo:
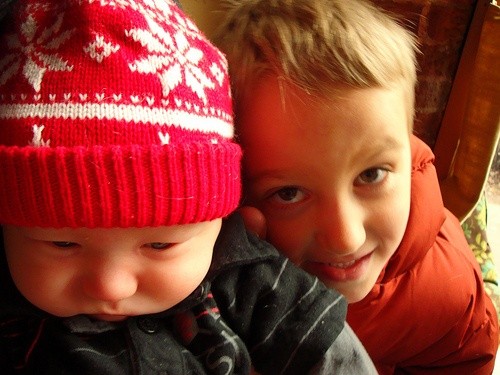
[0,0,377,375]
[210,0,500,374]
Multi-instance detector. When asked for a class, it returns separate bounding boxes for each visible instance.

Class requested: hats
[0,0,242,230]
